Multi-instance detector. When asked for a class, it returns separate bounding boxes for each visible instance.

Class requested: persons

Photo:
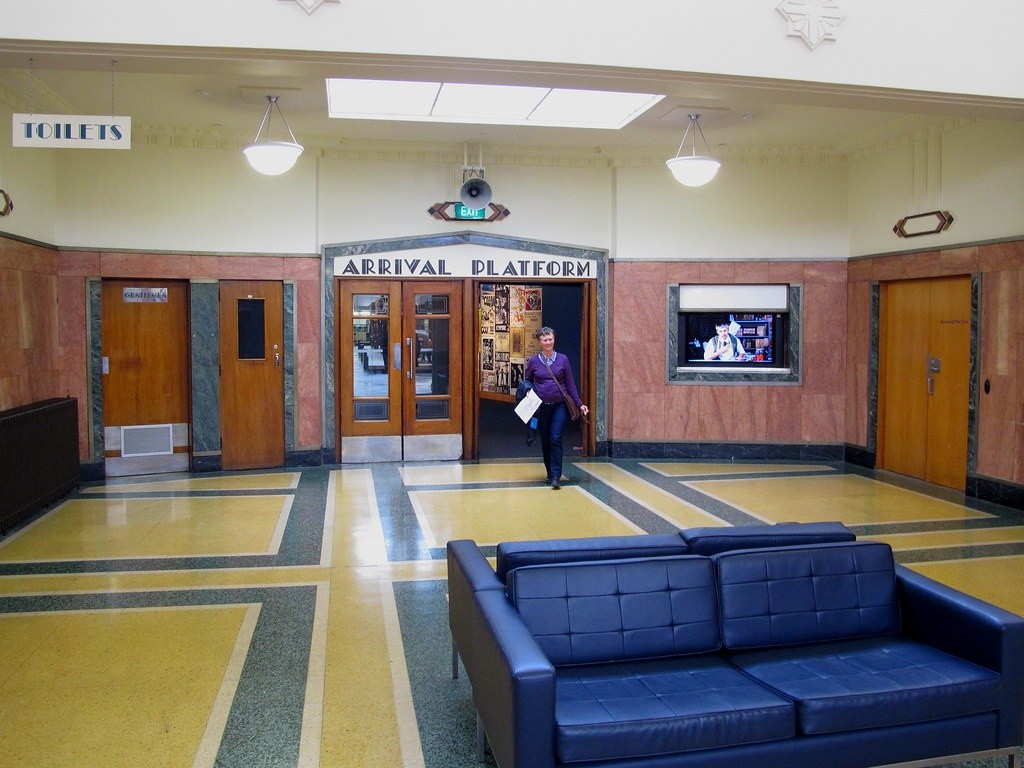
[704,320,747,361]
[495,364,508,386]
[525,328,589,488]
[511,364,524,387]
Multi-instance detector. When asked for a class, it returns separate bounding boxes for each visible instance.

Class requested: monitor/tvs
[678,312,784,368]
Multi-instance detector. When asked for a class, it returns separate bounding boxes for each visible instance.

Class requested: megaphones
[459,177,492,210]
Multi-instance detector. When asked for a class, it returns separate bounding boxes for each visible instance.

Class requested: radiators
[0,398,83,535]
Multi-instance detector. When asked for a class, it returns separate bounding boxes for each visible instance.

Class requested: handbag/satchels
[566,395,581,420]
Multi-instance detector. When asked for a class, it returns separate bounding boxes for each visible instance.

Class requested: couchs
[447,520,858,678]
[473,541,1024,768]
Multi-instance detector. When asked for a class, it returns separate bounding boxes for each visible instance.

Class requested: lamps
[242,96,304,176]
[665,114,723,187]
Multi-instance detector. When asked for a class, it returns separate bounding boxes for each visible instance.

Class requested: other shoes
[546,475,551,483]
[551,476,561,488]
